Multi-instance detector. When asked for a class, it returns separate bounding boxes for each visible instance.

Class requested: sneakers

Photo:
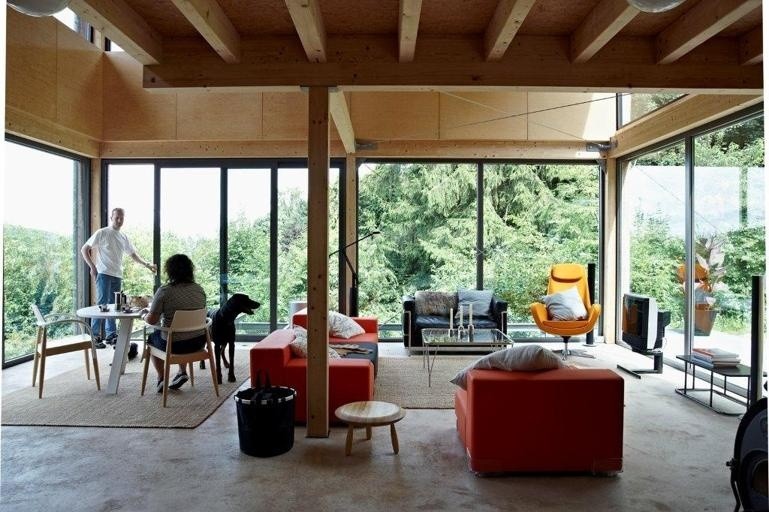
[93,336,106,348]
[157,377,163,392]
[168,373,188,389]
[106,335,118,345]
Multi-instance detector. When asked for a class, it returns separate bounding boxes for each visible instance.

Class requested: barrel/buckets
[234,386,299,459]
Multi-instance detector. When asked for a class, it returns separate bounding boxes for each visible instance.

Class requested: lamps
[6,1,69,18]
[627,1,685,15]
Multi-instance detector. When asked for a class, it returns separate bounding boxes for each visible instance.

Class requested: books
[691,347,741,368]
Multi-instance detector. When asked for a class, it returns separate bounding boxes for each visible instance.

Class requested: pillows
[328,310,365,339]
[289,323,340,358]
[539,286,588,321]
[454,288,493,319]
[450,345,564,392]
[414,291,457,316]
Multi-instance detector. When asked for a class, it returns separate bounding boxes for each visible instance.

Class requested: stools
[335,401,406,456]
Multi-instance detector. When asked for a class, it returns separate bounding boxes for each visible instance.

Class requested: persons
[139,253,206,391]
[81,207,157,349]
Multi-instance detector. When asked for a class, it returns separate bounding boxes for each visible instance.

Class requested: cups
[150,264,158,275]
[99,304,110,311]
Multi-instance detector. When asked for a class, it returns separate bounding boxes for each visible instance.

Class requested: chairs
[455,365,624,478]
[29,303,100,399]
[141,308,219,408]
[249,329,378,425]
[726,397,768,512]
[293,307,378,343]
[529,264,602,362]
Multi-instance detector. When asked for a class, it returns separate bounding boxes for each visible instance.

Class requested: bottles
[114,292,141,313]
[449,323,474,336]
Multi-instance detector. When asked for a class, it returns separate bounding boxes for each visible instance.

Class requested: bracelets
[145,262,150,266]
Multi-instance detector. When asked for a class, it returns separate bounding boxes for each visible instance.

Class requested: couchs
[402,289,508,357]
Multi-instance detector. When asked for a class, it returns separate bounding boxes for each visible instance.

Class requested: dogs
[199,293,259,384]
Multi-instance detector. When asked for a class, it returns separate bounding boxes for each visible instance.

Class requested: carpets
[372,356,482,409]
[8,349,251,429]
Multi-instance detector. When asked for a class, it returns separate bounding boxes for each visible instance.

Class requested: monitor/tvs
[622,293,671,350]
